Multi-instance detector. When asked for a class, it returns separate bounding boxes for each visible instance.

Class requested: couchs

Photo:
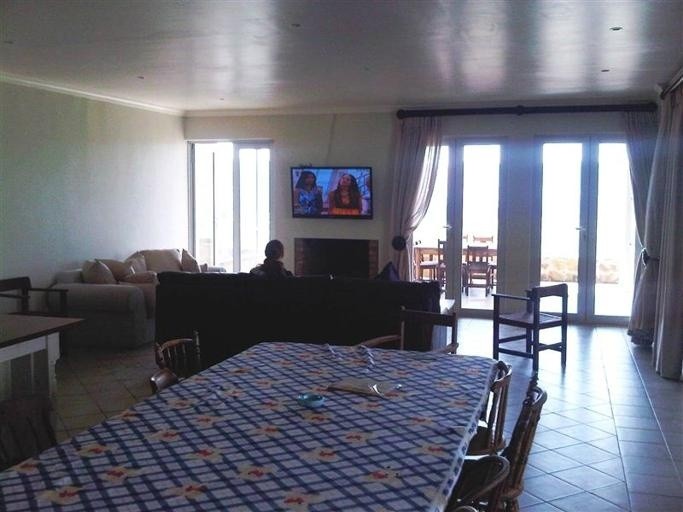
[152,271,443,372]
[45,247,226,352]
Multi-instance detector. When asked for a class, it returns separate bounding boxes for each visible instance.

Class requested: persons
[251,239,295,276]
[328,175,363,213]
[292,171,323,216]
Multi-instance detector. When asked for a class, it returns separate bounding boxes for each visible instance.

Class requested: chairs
[488,282,570,370]
[144,368,180,393]
[0,392,64,473]
[152,329,203,380]
[352,303,459,356]
[410,233,500,299]
[445,355,549,511]
[0,275,69,317]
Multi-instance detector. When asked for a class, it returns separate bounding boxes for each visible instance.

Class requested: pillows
[74,249,209,290]
[373,262,399,283]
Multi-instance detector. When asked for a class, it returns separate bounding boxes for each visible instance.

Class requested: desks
[0,341,501,511]
[0,314,85,442]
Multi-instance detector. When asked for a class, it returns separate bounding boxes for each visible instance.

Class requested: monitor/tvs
[290,166,373,221]
